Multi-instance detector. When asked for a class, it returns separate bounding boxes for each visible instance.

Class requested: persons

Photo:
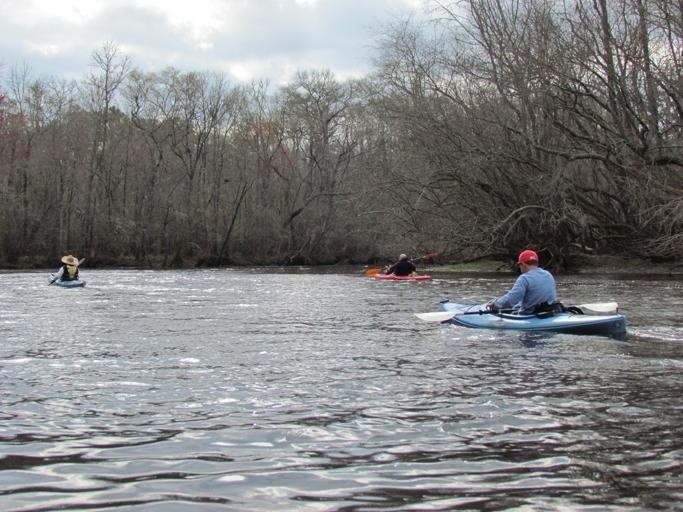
[486,250,556,315]
[52,255,80,282]
[386,254,416,276]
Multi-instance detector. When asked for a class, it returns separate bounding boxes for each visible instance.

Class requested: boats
[48,273,86,288]
[439,299,627,341]
[375,273,431,280]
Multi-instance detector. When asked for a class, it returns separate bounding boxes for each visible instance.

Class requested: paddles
[414,301,618,323]
[48,257,86,288]
[367,252,441,274]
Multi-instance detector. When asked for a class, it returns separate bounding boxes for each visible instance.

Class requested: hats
[398,254,407,261]
[515,250,538,268]
[61,255,79,265]
